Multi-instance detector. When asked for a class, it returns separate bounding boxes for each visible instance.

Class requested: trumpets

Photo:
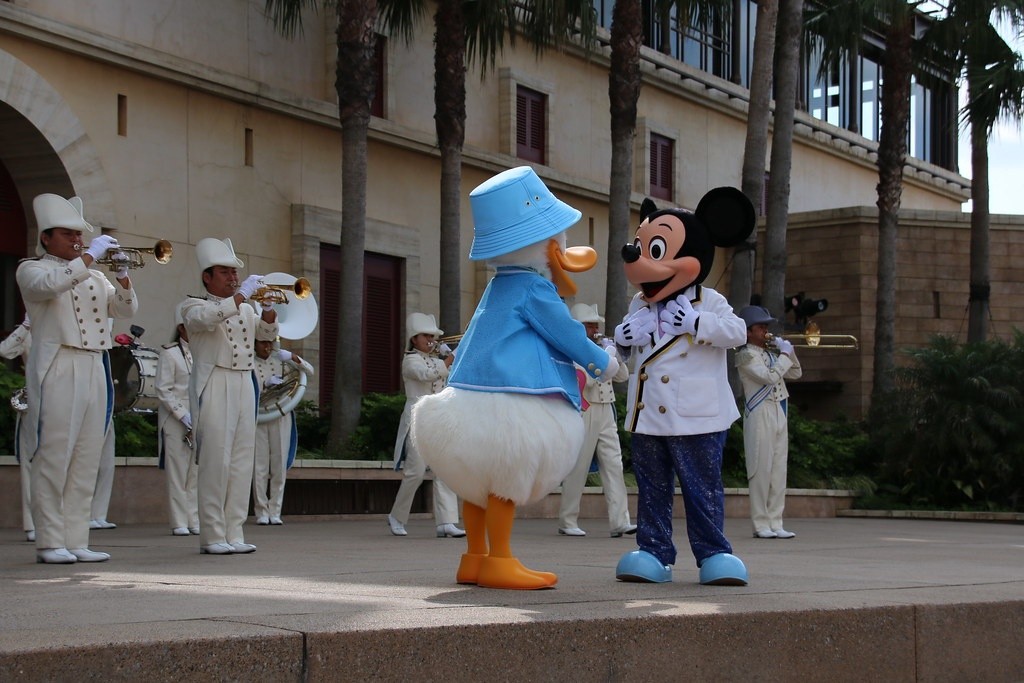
[424,335,463,349]
[73,238,173,271]
[593,332,614,352]
[230,276,310,304]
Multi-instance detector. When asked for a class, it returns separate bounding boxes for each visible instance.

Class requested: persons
[0,193,138,563]
[384,313,466,537]
[558,303,637,538]
[154,302,200,536]
[181,238,279,554]
[735,306,803,539]
[253,338,314,526]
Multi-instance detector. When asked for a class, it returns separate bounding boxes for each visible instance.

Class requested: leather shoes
[88,519,117,529]
[437,524,466,538]
[37,547,110,564]
[386,513,407,535]
[753,529,795,538]
[172,527,200,535]
[256,516,283,524]
[611,525,637,537]
[559,528,585,536]
[200,542,255,554]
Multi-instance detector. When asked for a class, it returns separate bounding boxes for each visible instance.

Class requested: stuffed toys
[410,166,619,590]
[615,186,756,586]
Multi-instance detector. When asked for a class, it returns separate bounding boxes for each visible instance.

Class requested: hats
[738,305,775,328]
[176,301,184,326]
[405,313,444,353]
[196,238,244,272]
[571,303,605,323]
[32,193,94,233]
[36,229,46,257]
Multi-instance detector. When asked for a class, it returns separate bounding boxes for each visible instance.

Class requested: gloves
[255,289,273,311]
[264,375,283,387]
[440,343,452,356]
[22,311,30,329]
[238,275,267,301]
[277,349,292,361]
[593,332,616,351]
[83,235,120,262]
[452,347,457,357]
[770,337,794,356]
[111,252,129,280]
[180,412,192,430]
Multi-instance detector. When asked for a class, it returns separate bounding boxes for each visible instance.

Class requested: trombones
[762,320,860,353]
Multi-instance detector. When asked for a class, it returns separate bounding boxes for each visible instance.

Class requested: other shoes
[26,530,36,541]
